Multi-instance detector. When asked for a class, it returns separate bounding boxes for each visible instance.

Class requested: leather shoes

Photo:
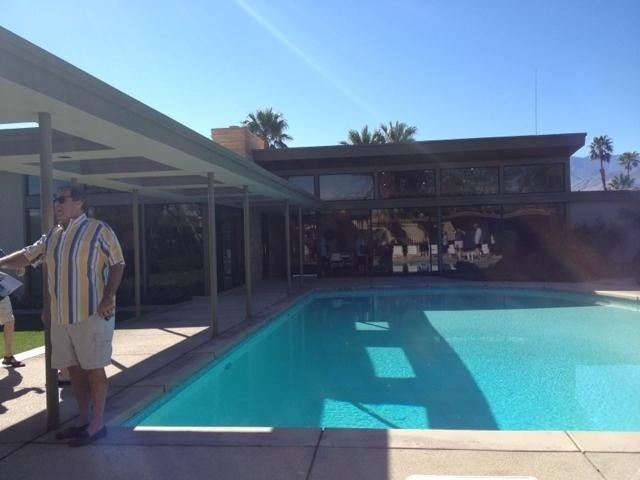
[68,425,107,447]
[56,423,89,439]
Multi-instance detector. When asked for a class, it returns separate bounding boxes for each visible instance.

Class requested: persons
[0,184,126,447]
[318,233,334,277]
[473,224,483,259]
[1,247,25,367]
[442,220,466,262]
[303,233,317,264]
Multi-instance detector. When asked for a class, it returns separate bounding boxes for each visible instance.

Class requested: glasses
[52,195,72,204]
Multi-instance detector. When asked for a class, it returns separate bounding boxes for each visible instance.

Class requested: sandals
[2,356,25,367]
